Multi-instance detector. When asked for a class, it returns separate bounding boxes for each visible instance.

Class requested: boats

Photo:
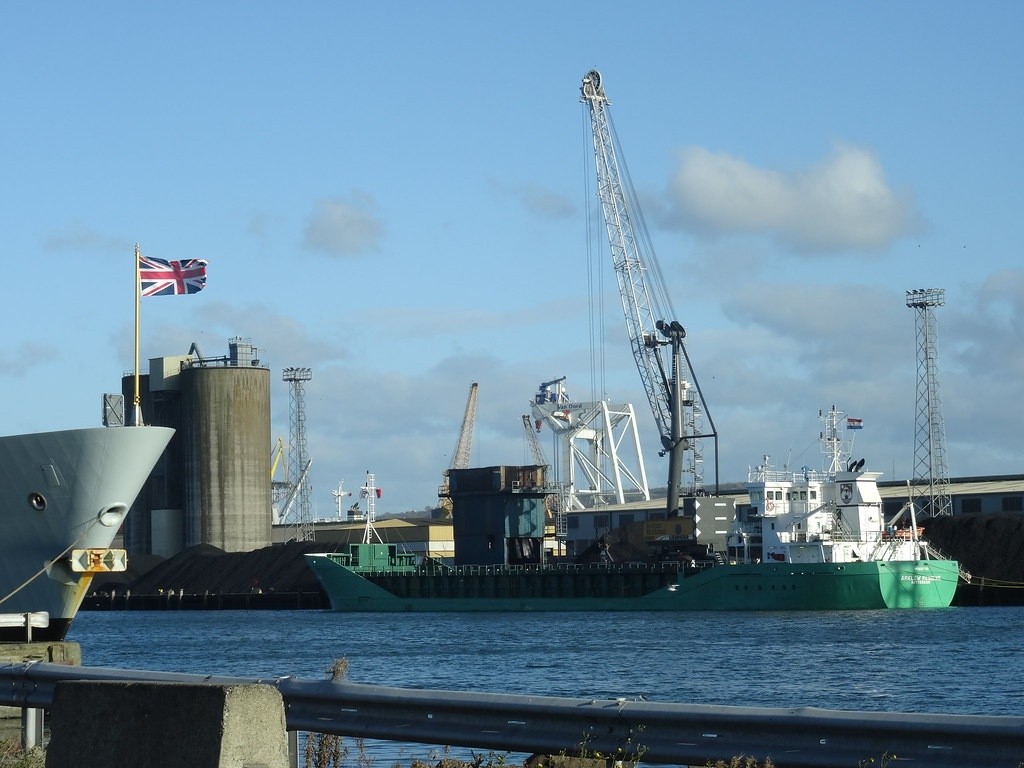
[302,321,959,611]
[1,406,174,644]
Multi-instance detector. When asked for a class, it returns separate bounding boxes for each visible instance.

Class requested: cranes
[576,68,721,502]
[521,414,554,519]
[433,383,479,520]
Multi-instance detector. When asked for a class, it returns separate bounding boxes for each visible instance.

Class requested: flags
[847,417,864,430]
[139,256,209,297]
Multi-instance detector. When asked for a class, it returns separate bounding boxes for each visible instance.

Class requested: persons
[598,544,614,563]
